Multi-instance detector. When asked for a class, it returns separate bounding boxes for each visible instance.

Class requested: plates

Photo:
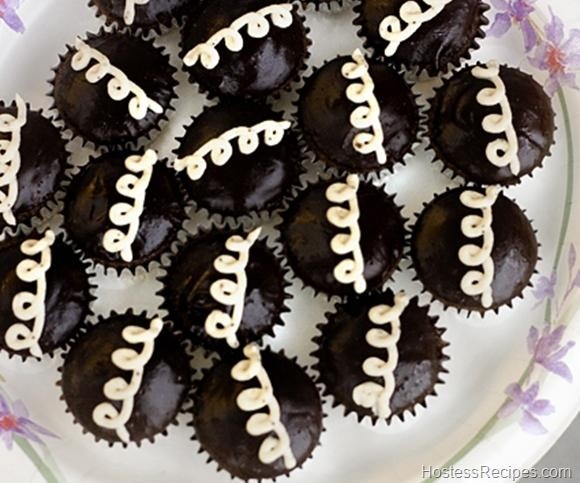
[0,1,578,483]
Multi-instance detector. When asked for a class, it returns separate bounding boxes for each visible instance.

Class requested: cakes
[0,0,558,483]
